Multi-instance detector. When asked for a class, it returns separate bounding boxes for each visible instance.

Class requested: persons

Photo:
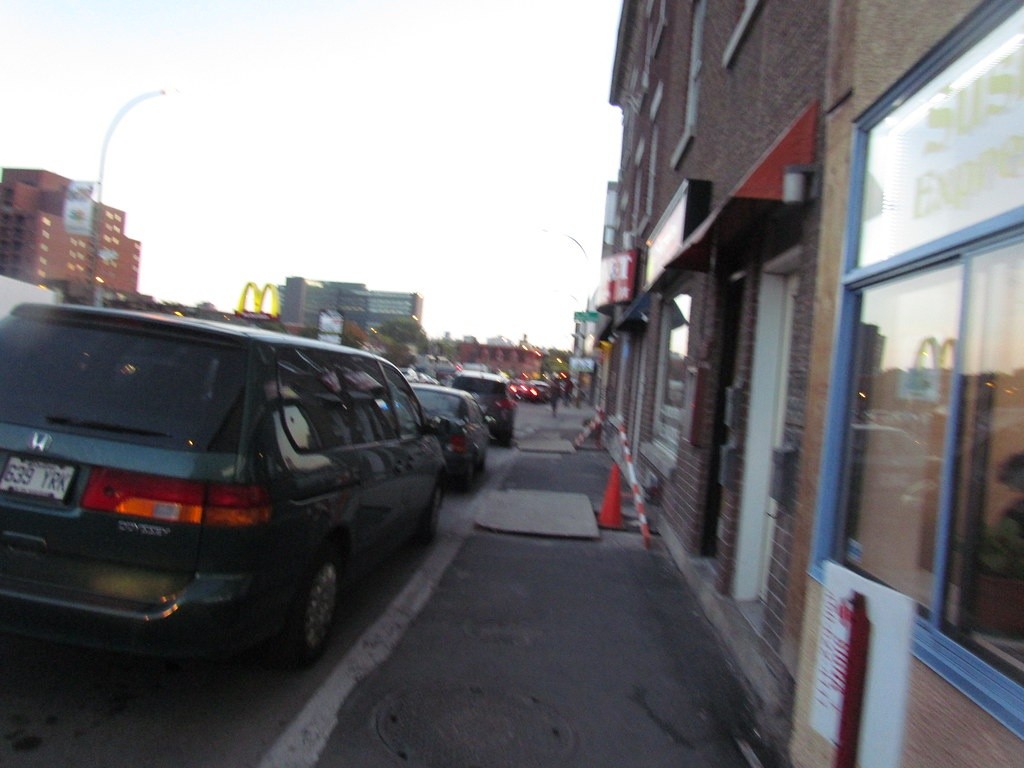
[563,378,574,405]
[998,450,1024,538]
[550,378,561,417]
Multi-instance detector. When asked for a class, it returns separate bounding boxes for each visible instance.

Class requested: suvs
[0,305,467,669]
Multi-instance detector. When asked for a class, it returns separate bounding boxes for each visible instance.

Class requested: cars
[452,370,521,447]
[510,378,554,405]
[404,384,497,481]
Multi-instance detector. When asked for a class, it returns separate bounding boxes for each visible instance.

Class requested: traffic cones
[595,464,627,530]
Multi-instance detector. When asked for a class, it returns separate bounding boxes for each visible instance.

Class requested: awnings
[646,95,819,293]
[593,291,649,348]
